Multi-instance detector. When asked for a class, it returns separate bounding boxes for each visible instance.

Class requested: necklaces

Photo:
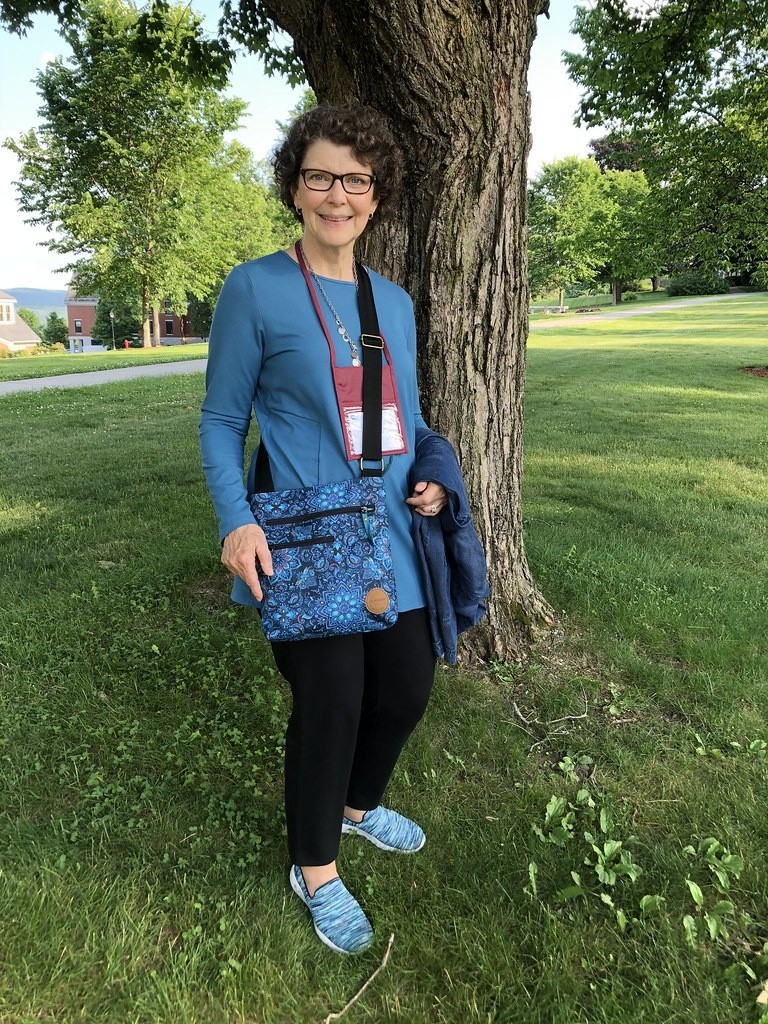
[299,238,361,367]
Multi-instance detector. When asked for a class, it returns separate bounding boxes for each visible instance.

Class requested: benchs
[530,306,569,314]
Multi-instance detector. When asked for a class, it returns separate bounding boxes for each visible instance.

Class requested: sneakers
[342,806,426,853]
[290,865,374,955]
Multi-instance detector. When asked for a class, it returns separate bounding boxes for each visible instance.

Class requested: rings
[431,504,437,513]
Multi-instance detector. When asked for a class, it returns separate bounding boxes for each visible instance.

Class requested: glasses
[300,168,376,195]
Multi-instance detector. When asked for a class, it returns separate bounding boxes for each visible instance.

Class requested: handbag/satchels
[250,476,397,641]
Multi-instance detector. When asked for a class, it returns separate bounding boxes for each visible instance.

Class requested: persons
[200,105,447,956]
